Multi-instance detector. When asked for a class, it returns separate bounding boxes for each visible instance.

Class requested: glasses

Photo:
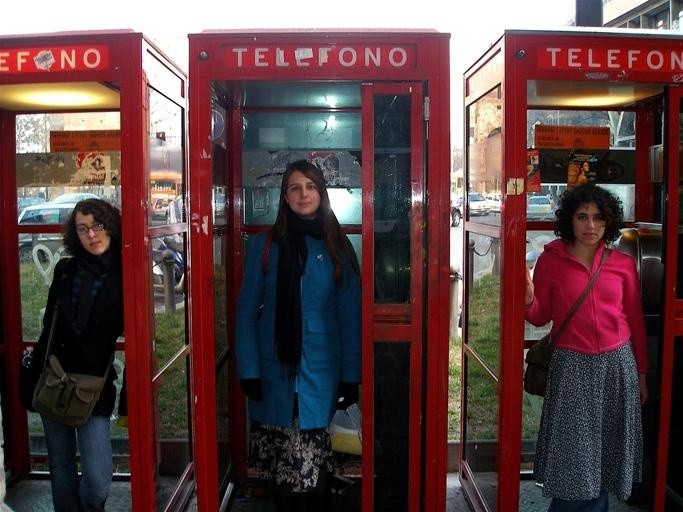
[77,221,105,236]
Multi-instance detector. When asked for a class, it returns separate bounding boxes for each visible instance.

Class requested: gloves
[239,378,264,402]
[335,382,359,411]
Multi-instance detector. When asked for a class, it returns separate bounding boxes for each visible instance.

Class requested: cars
[15,173,227,304]
[448,183,570,227]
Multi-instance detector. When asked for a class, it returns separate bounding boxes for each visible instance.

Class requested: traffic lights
[157,132,164,146]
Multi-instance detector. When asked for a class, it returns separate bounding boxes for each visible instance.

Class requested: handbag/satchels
[31,353,105,428]
[523,335,555,397]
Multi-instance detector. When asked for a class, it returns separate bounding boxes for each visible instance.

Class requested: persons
[579,160,595,183]
[19,195,133,512]
[522,184,648,511]
[232,158,360,511]
